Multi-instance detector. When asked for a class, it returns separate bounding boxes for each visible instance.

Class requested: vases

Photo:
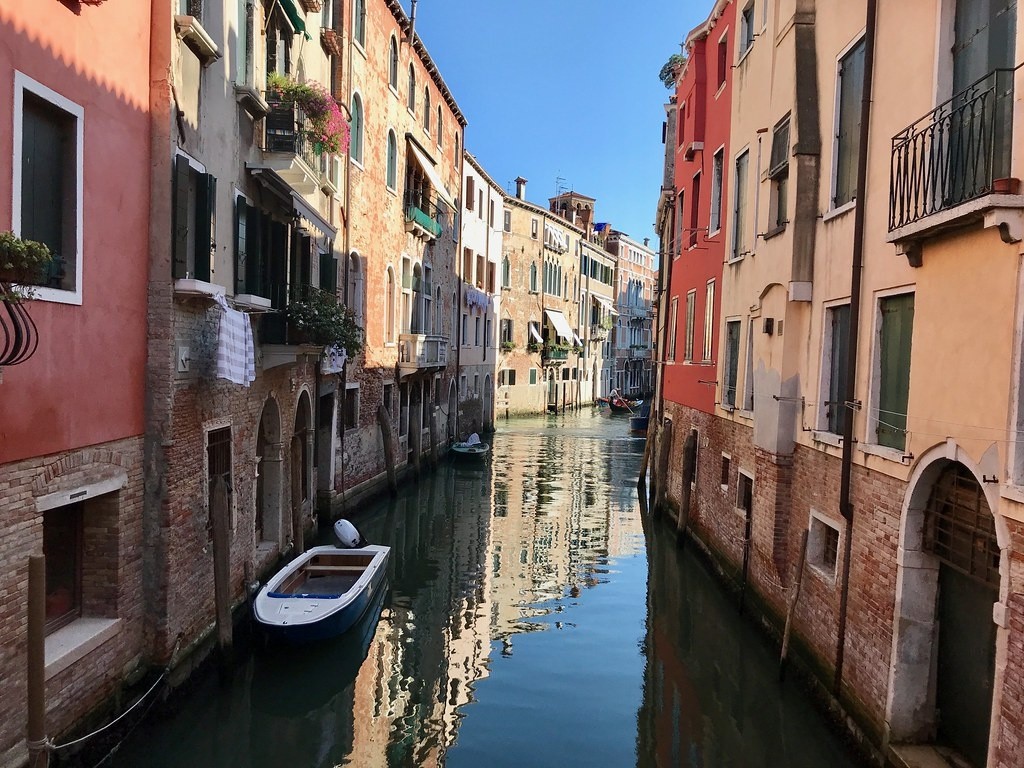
[993,178,1021,195]
[174,278,226,295]
[234,294,272,307]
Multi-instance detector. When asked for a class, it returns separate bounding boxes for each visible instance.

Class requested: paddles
[615,392,634,416]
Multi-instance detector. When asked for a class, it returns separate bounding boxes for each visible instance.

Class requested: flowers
[269,71,351,155]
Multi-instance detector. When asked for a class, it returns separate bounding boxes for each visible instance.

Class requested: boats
[250,518,391,643]
[608,396,644,413]
[452,433,490,460]
[596,397,628,407]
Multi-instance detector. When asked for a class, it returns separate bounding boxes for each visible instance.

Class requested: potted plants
[0,230,52,304]
[502,340,583,354]
[325,29,341,51]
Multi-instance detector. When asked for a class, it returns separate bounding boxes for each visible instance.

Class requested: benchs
[303,565,366,571]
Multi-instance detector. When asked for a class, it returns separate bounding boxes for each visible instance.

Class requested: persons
[609,388,618,405]
